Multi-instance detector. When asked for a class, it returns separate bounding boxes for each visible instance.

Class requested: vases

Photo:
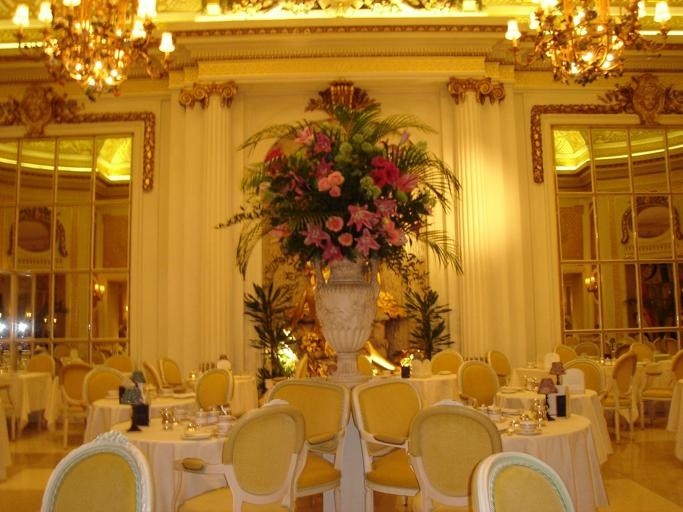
[304,260,385,388]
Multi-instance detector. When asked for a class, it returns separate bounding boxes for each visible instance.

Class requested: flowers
[216,97,476,293]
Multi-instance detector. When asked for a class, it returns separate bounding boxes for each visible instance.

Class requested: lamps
[9,1,178,94]
[504,2,671,87]
[584,273,597,294]
[92,279,105,305]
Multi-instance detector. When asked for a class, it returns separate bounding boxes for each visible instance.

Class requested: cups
[194,406,233,438]
[485,405,539,434]
